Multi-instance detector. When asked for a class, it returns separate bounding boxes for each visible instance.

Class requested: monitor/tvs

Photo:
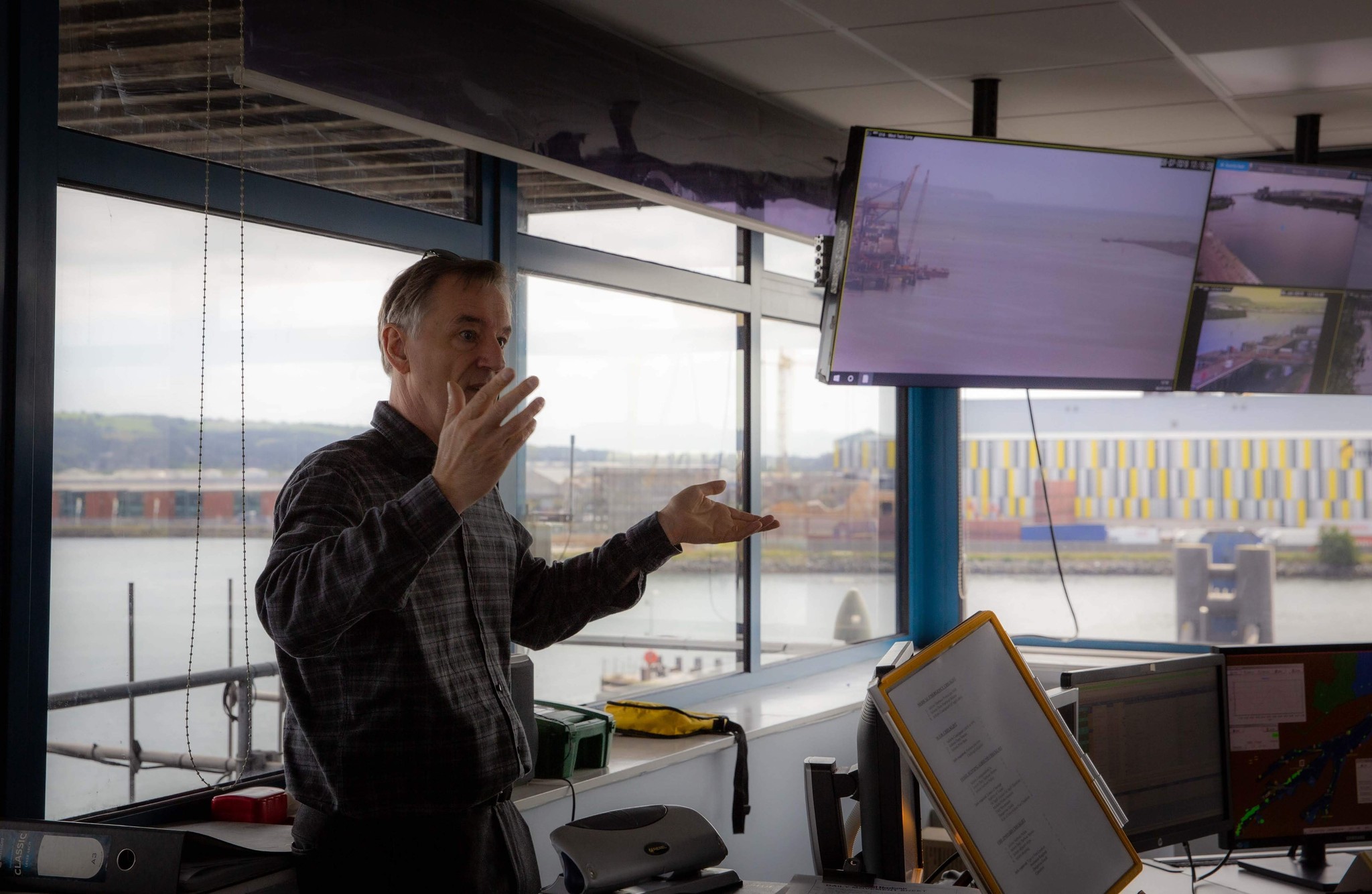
[804,611,1372,894]
[816,125,1372,395]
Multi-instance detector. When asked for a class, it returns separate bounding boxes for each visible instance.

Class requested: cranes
[777,352,794,475]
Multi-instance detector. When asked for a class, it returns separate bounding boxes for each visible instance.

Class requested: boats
[843,163,949,292]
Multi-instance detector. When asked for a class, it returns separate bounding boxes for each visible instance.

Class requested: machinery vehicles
[767,489,896,526]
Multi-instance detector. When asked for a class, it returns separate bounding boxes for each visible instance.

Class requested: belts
[451,784,513,823]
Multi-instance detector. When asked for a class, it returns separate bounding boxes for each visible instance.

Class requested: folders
[1,820,296,894]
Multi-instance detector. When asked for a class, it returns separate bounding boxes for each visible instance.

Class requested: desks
[161,624,1246,894]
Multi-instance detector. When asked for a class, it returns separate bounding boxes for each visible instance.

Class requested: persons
[255,248,781,894]
